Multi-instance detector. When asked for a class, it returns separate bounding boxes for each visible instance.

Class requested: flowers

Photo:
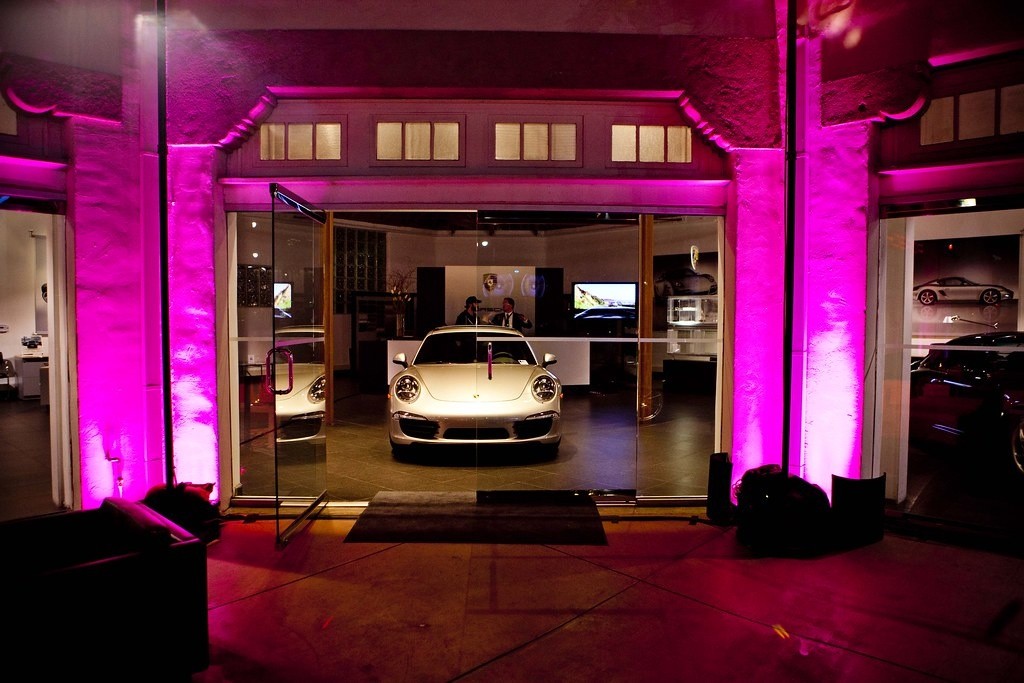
[380,256,428,336]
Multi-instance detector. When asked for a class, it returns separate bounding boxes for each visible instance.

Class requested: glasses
[502,303,510,305]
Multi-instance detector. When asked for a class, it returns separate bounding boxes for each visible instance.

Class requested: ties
[506,314,509,328]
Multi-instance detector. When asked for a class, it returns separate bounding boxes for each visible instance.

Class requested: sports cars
[913,276,1015,305]
[387,323,563,462]
[909,330,1024,483]
[274,324,326,437]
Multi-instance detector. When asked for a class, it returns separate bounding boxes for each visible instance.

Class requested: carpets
[342,491,609,546]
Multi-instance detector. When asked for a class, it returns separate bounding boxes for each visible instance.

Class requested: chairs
[0,496,210,683]
[434,336,464,362]
[0,352,19,396]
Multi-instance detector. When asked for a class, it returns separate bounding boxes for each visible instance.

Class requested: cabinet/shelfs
[15,355,49,400]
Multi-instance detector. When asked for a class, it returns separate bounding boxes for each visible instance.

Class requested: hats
[466,296,482,304]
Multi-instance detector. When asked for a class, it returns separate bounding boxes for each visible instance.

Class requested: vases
[392,290,410,336]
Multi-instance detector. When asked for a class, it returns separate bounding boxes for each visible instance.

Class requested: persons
[489,298,533,334]
[457,296,482,324]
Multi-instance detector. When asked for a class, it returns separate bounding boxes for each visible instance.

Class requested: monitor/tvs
[273,283,294,310]
[570,282,639,312]
